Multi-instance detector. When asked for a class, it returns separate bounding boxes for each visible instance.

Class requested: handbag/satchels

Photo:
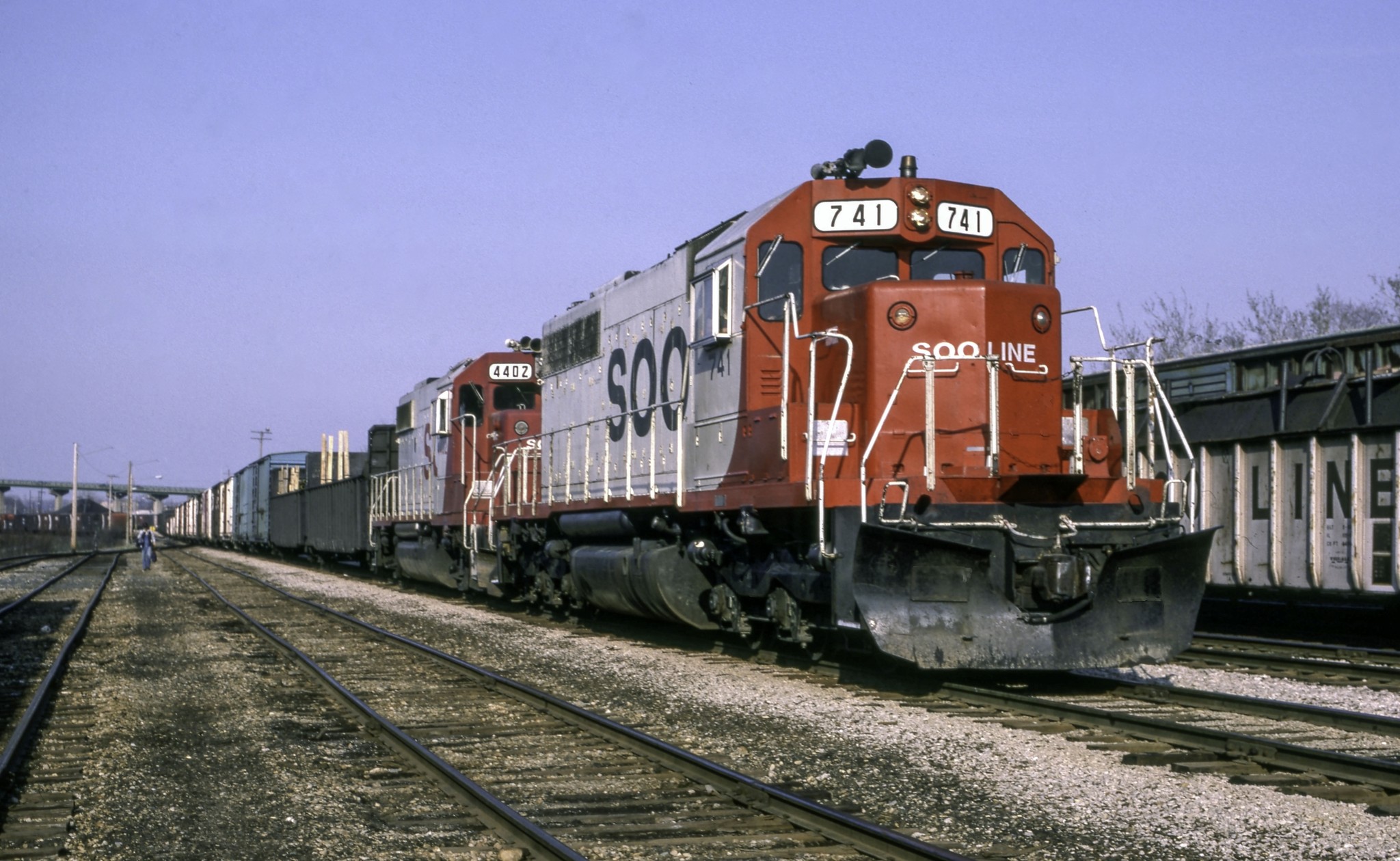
[151,548,157,562]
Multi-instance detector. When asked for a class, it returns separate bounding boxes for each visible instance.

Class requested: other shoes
[147,567,150,570]
[142,569,146,573]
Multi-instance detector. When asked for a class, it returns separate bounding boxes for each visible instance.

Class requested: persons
[137,523,156,573]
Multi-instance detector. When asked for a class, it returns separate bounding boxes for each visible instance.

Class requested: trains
[161,138,1226,668]
